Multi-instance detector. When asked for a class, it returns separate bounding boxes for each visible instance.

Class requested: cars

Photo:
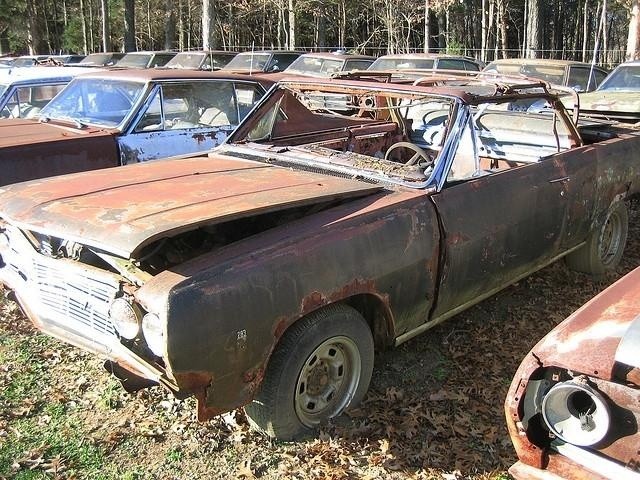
[0,50,640,187]
[1,67,640,446]
[504,265,640,480]
